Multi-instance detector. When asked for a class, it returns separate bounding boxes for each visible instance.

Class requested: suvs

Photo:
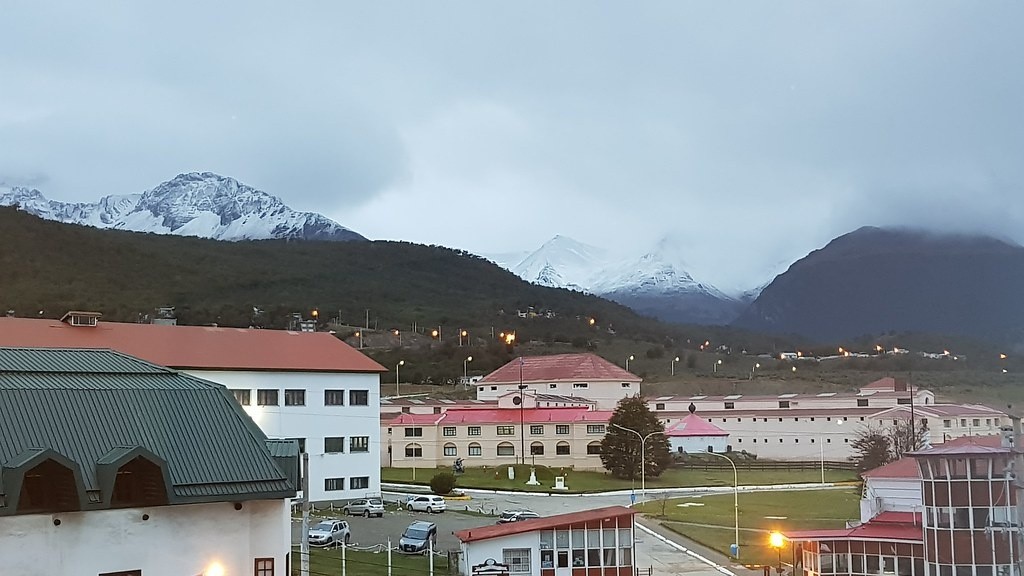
[342,498,384,517]
[398,521,437,554]
[309,519,352,546]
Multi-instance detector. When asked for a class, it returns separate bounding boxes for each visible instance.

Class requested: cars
[406,496,447,513]
[496,511,540,525]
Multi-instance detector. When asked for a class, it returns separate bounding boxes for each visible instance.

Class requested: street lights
[672,357,680,376]
[771,533,784,576]
[698,448,739,560]
[612,423,673,506]
[464,357,472,391]
[626,353,634,372]
[821,434,829,483]
[396,360,405,398]
[399,413,417,480]
